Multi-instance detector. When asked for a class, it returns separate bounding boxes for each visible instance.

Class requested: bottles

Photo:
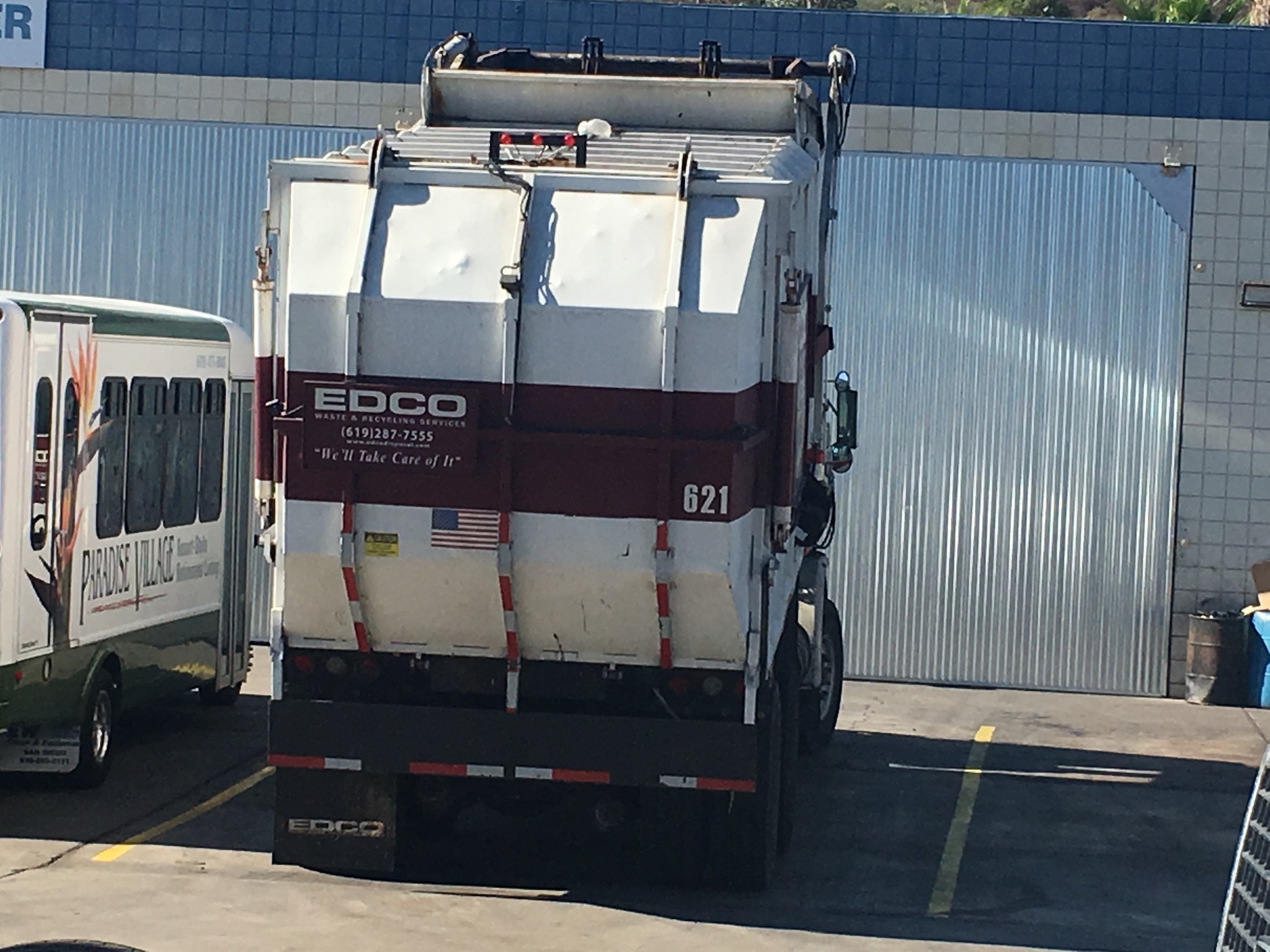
[577,118,613,137]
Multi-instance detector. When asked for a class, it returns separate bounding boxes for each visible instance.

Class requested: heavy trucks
[237,33,858,891]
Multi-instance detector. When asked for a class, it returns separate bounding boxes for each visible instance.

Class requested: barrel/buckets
[1242,608,1270,709]
[1184,610,1245,705]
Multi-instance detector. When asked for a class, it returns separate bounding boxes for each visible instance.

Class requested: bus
[1,291,256,794]
